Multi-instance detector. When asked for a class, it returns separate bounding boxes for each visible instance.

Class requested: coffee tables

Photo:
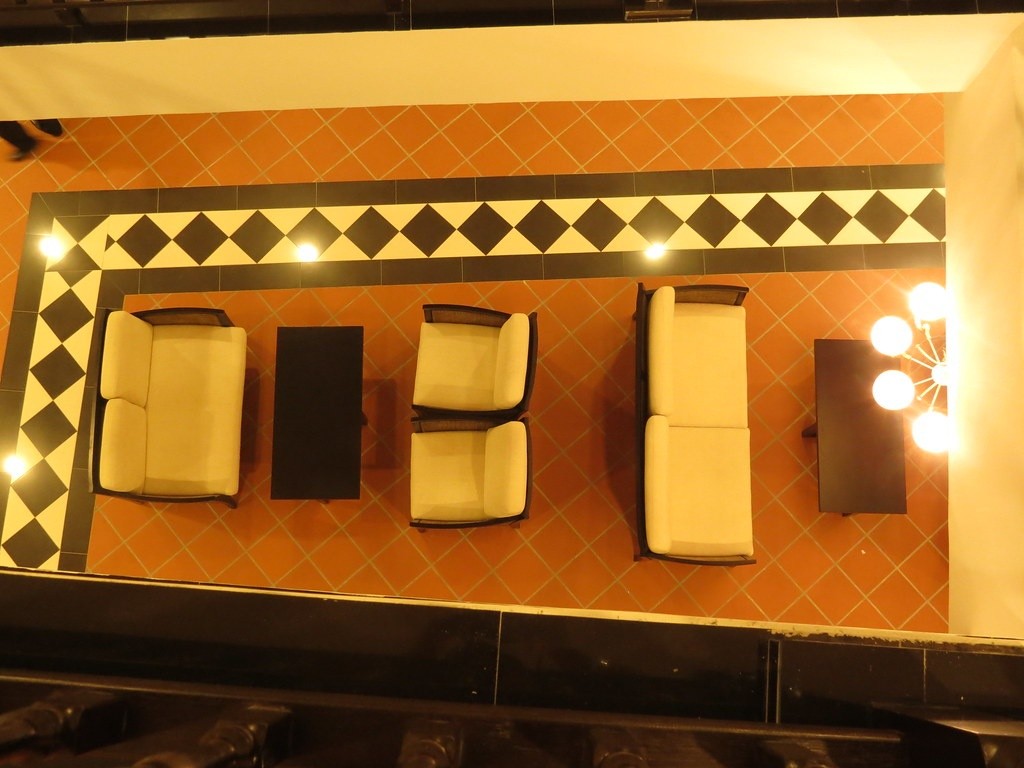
[801,339,907,517]
[271,327,368,504]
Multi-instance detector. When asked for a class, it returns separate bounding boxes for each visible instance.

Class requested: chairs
[410,304,538,532]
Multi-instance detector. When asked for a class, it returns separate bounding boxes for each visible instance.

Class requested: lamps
[870,281,947,454]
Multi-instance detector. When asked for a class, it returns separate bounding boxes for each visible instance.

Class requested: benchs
[87,307,246,509]
[632,282,757,567]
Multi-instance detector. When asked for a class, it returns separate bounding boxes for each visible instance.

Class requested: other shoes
[9,136,37,159]
[30,119,63,137]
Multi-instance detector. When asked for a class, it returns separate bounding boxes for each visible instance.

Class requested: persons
[0,119,64,161]
